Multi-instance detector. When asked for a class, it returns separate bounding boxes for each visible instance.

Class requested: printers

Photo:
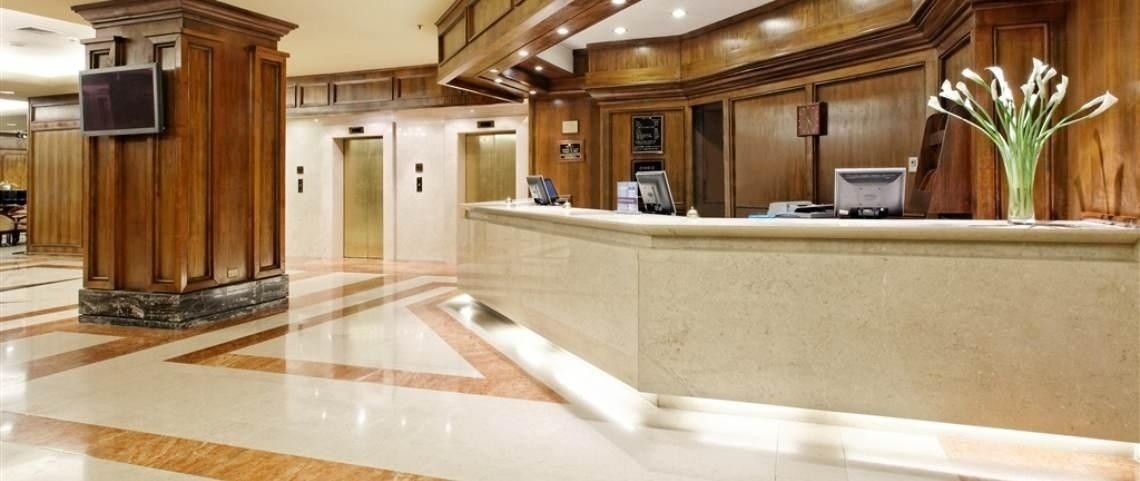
[767,200,835,218]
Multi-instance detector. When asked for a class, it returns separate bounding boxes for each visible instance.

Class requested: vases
[998,145,1041,224]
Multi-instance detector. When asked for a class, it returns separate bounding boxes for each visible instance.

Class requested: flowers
[928,57,1120,215]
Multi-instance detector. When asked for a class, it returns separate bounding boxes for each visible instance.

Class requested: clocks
[796,103,825,138]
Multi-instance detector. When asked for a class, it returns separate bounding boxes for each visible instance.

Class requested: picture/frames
[556,140,586,163]
[630,116,665,199]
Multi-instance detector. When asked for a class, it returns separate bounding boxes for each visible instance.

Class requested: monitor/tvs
[544,178,559,202]
[834,167,907,217]
[526,175,552,206]
[78,63,164,136]
[635,170,677,214]
